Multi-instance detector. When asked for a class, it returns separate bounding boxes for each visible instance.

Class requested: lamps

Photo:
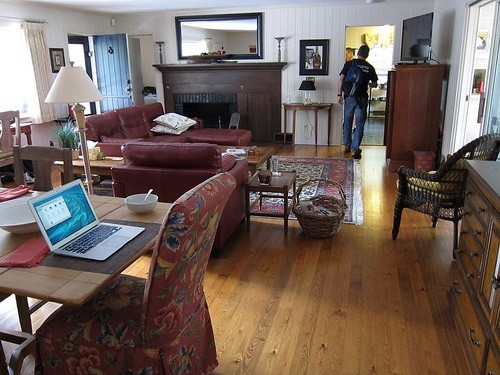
[299,81,317,105]
[44,61,105,195]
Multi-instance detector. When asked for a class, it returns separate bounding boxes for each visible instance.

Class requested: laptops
[27,179,145,261]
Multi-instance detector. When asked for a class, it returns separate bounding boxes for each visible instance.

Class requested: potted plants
[49,117,81,160]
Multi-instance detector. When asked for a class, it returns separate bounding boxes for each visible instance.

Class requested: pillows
[150,113,197,135]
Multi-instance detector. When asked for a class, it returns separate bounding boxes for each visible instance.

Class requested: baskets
[292,178,347,238]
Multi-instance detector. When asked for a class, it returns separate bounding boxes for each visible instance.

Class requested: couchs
[85,102,251,156]
[110,143,249,257]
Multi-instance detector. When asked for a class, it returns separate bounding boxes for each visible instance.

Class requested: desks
[0,187,174,338]
[11,123,32,145]
[50,155,124,191]
[283,103,333,148]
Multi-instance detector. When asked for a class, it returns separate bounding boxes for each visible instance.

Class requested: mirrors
[175,13,263,59]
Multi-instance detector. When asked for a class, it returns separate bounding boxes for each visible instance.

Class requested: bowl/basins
[124,194,158,214]
[0,197,41,234]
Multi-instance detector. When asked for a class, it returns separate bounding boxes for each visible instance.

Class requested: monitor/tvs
[397,12,434,64]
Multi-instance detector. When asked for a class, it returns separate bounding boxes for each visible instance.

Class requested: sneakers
[352,149,363,158]
[343,146,351,153]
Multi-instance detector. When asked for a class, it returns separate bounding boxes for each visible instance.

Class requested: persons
[345,47,355,62]
[337,44,378,159]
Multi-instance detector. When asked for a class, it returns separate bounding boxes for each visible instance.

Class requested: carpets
[249,155,364,226]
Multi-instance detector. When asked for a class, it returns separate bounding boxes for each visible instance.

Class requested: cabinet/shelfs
[447,159,500,375]
[152,62,287,142]
[386,64,450,173]
[368,84,388,118]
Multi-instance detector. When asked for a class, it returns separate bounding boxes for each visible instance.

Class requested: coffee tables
[220,146,275,176]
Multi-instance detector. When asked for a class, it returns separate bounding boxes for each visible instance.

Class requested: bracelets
[338,95,342,96]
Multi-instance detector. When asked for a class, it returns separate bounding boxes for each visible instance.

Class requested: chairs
[0,110,237,375]
[390,133,500,240]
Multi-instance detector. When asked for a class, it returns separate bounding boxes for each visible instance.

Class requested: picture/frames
[49,48,65,73]
[300,39,329,75]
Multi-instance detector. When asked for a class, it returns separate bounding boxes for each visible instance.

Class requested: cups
[270,157,279,173]
[248,150,255,157]
[258,170,272,185]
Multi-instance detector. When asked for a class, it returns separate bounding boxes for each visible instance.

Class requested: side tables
[245,170,297,239]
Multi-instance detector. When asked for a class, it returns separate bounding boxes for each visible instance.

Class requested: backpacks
[342,59,367,97]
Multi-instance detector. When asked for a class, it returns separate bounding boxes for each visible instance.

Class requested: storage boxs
[413,151,436,172]
